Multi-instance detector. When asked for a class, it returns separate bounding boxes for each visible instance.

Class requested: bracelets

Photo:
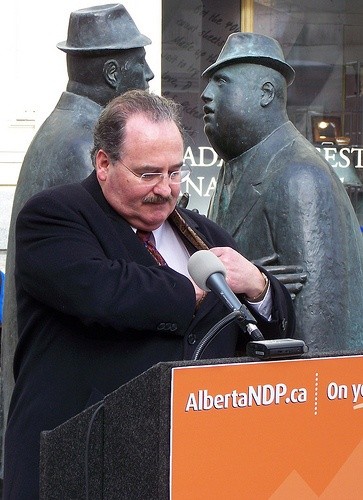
[197,291,208,305]
[248,273,269,301]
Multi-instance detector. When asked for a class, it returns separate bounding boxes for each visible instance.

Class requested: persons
[2,90,296,500]
[178,32,363,356]
[2,3,307,500]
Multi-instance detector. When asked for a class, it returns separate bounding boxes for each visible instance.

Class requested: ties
[136,229,168,267]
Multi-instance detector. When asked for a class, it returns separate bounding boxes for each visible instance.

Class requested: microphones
[188,250,264,341]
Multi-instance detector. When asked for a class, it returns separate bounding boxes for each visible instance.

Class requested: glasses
[112,153,193,185]
